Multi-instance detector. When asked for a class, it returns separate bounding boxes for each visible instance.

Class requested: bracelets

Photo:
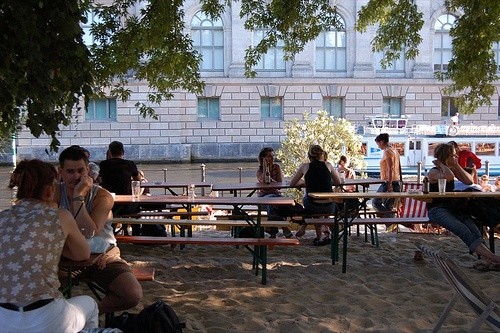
[72,195,85,201]
[388,181,392,186]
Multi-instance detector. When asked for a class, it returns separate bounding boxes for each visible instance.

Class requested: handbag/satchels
[140,224,168,237]
[233,207,261,238]
[112,301,183,333]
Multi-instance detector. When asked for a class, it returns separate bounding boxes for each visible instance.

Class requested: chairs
[412,241,500,333]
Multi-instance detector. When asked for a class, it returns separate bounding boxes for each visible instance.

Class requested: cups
[187,184,195,200]
[131,181,140,201]
[438,179,446,195]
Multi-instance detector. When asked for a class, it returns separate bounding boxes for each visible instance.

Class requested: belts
[0,298,54,311]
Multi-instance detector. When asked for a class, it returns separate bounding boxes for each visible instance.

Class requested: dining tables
[58,244,115,304]
[308,190,499,275]
[211,172,388,237]
[136,180,213,236]
[108,193,295,276]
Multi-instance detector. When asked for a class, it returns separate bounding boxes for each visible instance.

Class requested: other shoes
[478,262,500,271]
[384,223,397,234]
[285,231,293,238]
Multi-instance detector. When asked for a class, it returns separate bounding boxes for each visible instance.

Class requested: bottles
[265,167,271,184]
[423,169,430,194]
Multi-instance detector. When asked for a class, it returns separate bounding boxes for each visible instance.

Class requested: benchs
[71,261,154,330]
[120,210,209,251]
[303,217,500,266]
[215,209,397,250]
[139,205,194,247]
[113,233,300,286]
[211,206,371,237]
[107,216,290,270]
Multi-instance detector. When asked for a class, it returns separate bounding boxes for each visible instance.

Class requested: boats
[354,114,500,176]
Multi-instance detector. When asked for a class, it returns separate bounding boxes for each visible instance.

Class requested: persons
[371,132,403,233]
[52,145,143,317]
[321,149,332,240]
[97,140,144,235]
[80,146,100,186]
[289,144,341,246]
[257,146,301,245]
[421,142,500,268]
[335,154,356,218]
[447,140,482,172]
[389,141,405,155]
[457,142,471,150]
[0,157,100,332]
[428,142,443,155]
[106,149,133,235]
[479,174,495,193]
[475,141,495,155]
[194,207,268,232]
[345,142,368,156]
[492,176,500,191]
[137,169,151,196]
